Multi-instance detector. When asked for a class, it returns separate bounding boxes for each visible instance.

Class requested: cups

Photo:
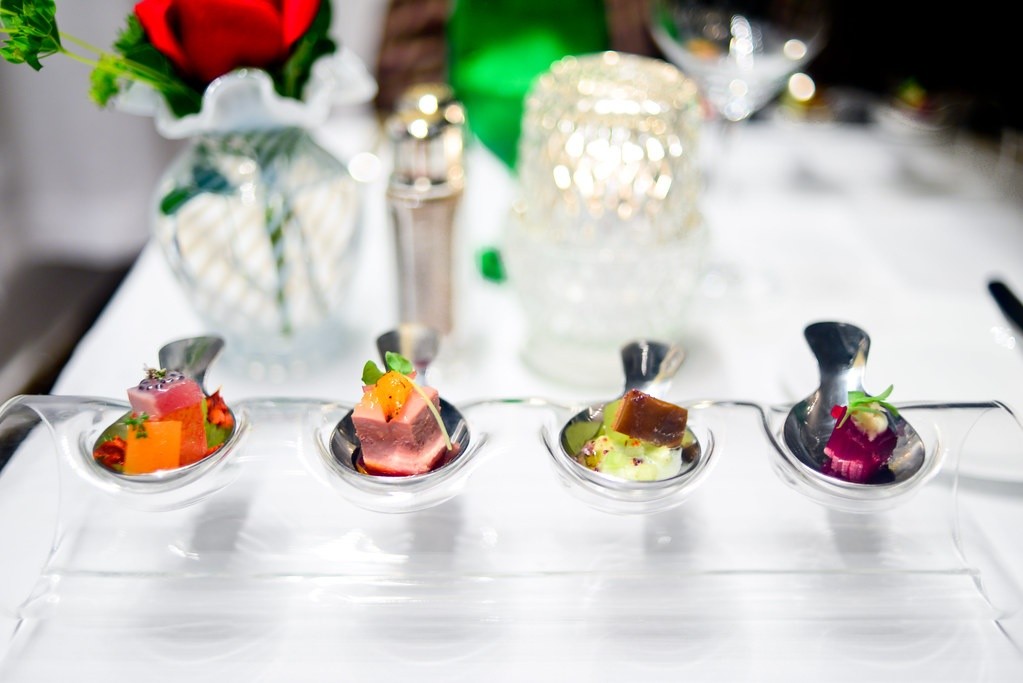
[650,0,829,120]
[498,51,718,387]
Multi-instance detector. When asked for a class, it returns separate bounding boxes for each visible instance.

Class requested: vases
[116,52,377,386]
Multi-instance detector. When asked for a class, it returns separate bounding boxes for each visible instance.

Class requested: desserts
[105,350,909,484]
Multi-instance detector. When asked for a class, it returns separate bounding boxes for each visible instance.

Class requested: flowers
[0,0,343,325]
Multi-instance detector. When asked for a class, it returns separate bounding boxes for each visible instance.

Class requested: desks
[0,87,1023,683]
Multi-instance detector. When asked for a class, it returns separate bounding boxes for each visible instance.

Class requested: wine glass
[448,1,609,281]
